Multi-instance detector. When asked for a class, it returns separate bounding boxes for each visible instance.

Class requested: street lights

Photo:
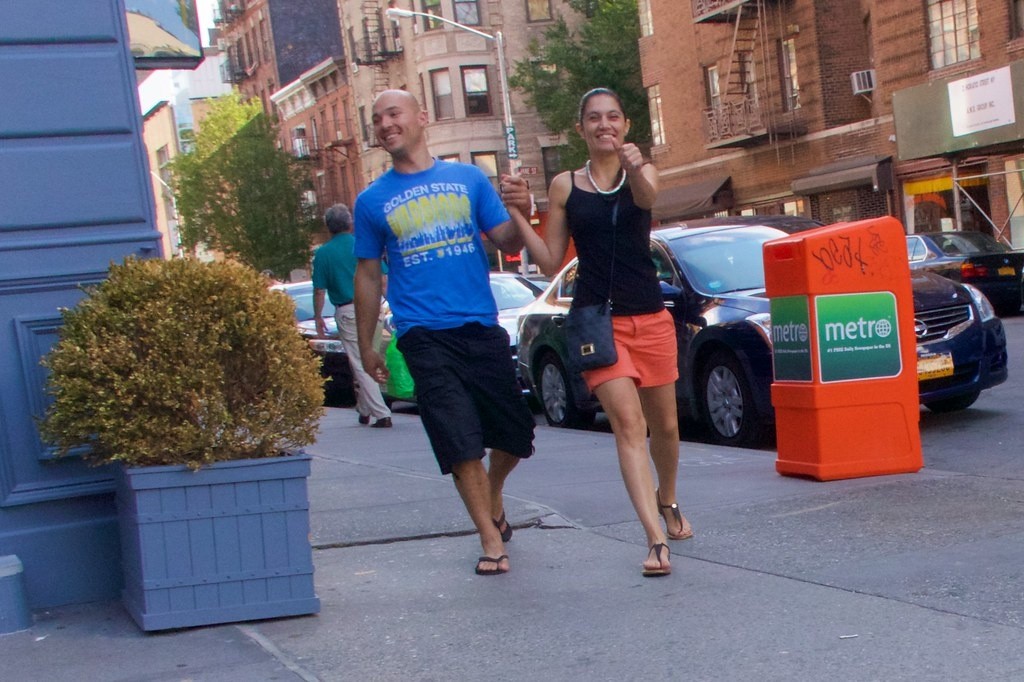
[385,5,523,176]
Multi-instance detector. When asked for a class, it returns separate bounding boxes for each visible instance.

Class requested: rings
[525,181,529,189]
[500,184,503,192]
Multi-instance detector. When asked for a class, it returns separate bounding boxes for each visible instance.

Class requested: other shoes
[359,413,370,424]
[371,417,392,427]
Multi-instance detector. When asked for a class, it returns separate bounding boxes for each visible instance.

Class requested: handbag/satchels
[563,303,618,372]
[385,329,415,401]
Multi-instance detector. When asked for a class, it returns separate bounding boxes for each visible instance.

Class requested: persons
[498,88,693,575]
[311,203,392,428]
[355,90,531,575]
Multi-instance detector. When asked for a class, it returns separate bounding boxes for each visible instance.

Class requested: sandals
[642,543,672,577]
[656,487,694,539]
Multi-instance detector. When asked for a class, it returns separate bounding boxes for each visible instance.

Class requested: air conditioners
[849,69,875,95]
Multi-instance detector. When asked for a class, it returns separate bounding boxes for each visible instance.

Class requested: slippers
[491,508,513,543]
[475,554,509,575]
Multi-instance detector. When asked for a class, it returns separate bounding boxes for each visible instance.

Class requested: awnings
[650,176,729,220]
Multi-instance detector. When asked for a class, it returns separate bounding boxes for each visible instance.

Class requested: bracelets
[313,315,321,318]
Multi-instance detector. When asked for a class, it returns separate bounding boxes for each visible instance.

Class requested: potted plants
[35,252,334,634]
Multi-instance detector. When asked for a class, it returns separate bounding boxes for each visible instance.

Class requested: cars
[904,229,1024,315]
[268,267,556,416]
[511,213,1010,448]
